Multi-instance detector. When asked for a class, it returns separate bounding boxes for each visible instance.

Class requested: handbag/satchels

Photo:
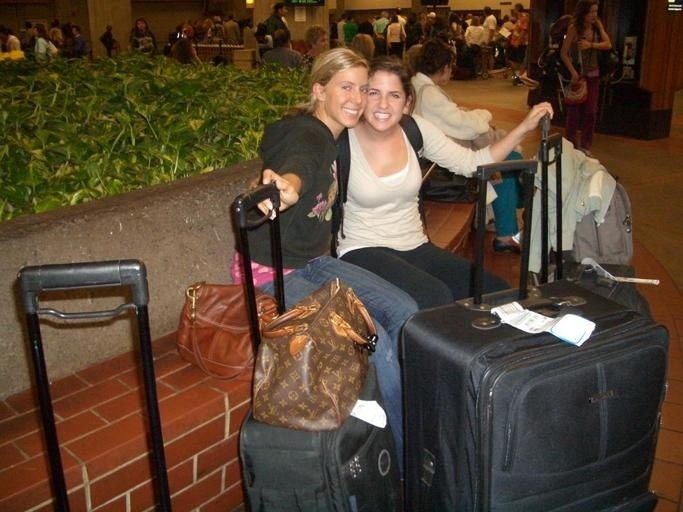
[177,276,280,381]
[251,278,377,432]
[560,24,588,105]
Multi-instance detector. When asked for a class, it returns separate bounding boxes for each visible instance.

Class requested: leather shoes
[494,238,523,254]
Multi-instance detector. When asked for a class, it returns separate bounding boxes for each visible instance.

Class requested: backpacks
[538,16,599,82]
[528,134,633,286]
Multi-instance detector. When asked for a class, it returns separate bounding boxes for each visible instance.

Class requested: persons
[48,21,63,47]
[394,7,405,28]
[343,15,358,45]
[510,17,516,26]
[232,49,418,484]
[265,3,287,51]
[177,23,193,44]
[170,38,196,65]
[464,17,485,51]
[481,6,496,70]
[404,13,420,50]
[99,26,115,59]
[0,26,19,53]
[130,18,155,55]
[386,15,405,60]
[255,24,265,44]
[504,4,529,86]
[61,24,75,59]
[362,17,374,36]
[501,15,514,35]
[206,17,223,44]
[559,1,612,158]
[372,12,389,54]
[336,55,554,309]
[336,15,346,46]
[410,41,532,252]
[20,22,34,51]
[71,25,84,57]
[302,25,329,72]
[465,14,471,27]
[350,34,373,61]
[328,19,340,40]
[260,29,301,70]
[225,13,239,45]
[31,23,52,58]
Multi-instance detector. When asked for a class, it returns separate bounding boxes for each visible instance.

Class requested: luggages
[231,184,400,512]
[537,133,654,326]
[540,105,631,267]
[401,158,671,512]
[17,259,171,512]
